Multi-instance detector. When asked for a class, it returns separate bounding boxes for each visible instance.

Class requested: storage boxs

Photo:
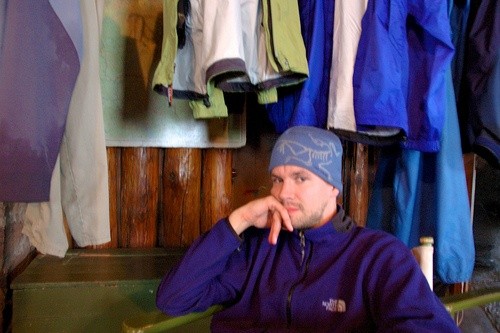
[10,248,213,333]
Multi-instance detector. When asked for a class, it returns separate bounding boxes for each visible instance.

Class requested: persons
[156,125,461,333]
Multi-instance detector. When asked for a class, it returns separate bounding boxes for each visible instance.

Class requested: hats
[269,125,343,196]
